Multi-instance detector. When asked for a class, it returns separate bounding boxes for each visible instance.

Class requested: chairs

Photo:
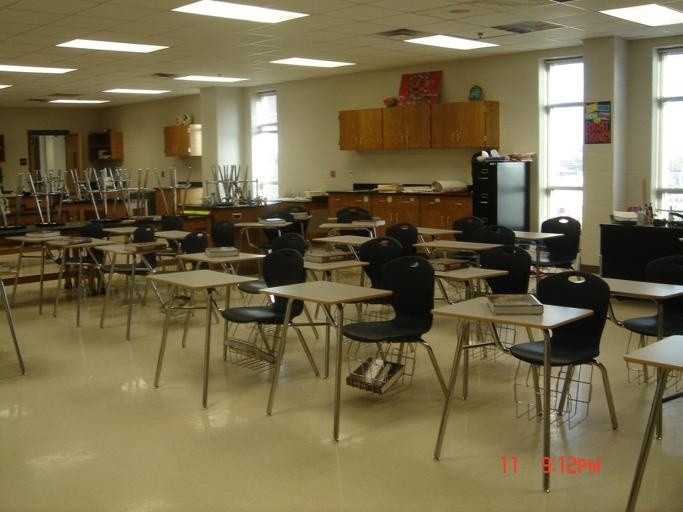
[56,207,683,430]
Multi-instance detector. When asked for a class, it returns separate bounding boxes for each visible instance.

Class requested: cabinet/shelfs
[420,197,473,252]
[339,107,382,149]
[327,192,373,234]
[374,193,420,236]
[165,124,202,157]
[431,101,499,148]
[473,161,530,250]
[382,102,431,149]
[88,132,123,159]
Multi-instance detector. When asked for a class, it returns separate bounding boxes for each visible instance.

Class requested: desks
[0,272,26,374]
[622,335,683,511]
[48,238,115,326]
[431,298,593,492]
[6,236,70,314]
[260,281,394,442]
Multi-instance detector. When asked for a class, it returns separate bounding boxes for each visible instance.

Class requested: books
[26,230,61,238]
[206,247,239,258]
[487,293,544,315]
[351,218,385,227]
[426,257,470,272]
[223,340,277,364]
[346,356,406,395]
[304,251,354,263]
[159,296,193,316]
[124,241,167,253]
[47,236,93,247]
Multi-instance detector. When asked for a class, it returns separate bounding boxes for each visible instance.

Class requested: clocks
[469,85,484,101]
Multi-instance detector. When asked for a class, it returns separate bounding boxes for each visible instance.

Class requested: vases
[386,98,397,107]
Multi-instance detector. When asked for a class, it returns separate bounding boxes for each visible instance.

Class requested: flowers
[384,96,392,104]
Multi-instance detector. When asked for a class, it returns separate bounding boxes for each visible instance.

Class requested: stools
[0,165,250,231]
